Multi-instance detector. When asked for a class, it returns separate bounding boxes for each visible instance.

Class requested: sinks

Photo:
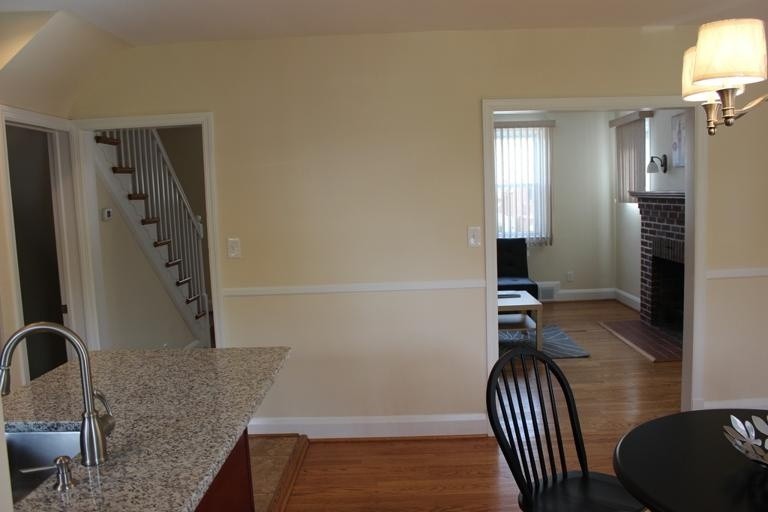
[4,420,81,508]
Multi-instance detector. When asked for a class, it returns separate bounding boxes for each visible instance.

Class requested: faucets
[0,322,95,412]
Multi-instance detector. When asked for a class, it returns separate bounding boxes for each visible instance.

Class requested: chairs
[485,347,647,511]
[497,238,539,317]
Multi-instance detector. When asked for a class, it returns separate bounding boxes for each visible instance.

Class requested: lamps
[646,155,668,174]
[681,17,767,136]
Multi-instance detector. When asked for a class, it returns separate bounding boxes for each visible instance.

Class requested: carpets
[598,317,682,363]
[498,325,589,359]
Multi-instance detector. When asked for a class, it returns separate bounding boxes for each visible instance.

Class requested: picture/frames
[671,113,685,167]
